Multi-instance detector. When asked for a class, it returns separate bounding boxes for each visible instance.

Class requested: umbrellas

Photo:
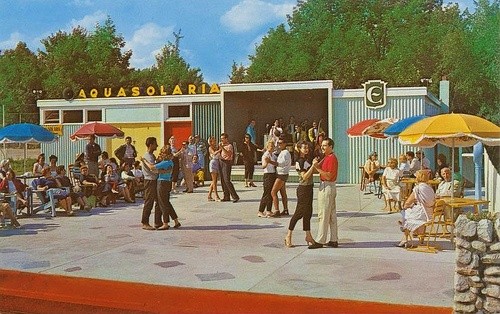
[346,118,380,153]
[0,122,58,184]
[383,114,433,170]
[399,111,500,249]
[362,118,402,169]
[69,122,125,143]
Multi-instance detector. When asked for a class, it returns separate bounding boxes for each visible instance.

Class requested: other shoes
[387,209,399,213]
[84,205,93,212]
[169,189,179,194]
[182,188,194,193]
[142,224,163,230]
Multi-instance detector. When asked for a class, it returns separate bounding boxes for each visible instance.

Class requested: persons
[209,133,240,203]
[362,149,461,232]
[86,134,101,178]
[239,133,264,187]
[208,137,223,201]
[141,137,173,230]
[258,139,277,218]
[246,119,260,165]
[265,138,291,217]
[140,145,181,230]
[395,170,436,248]
[264,116,328,166]
[165,134,212,194]
[114,137,137,167]
[285,140,319,247]
[308,138,338,249]
[0,151,144,229]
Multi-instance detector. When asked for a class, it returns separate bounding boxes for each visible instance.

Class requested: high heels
[12,223,22,229]
[125,197,136,203]
[393,240,413,248]
[280,209,289,215]
[305,238,316,245]
[99,202,108,207]
[244,183,257,187]
[284,237,294,247]
[66,210,74,216]
[271,210,280,217]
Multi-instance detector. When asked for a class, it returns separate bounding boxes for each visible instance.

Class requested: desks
[402,178,441,206]
[16,174,42,185]
[439,197,491,238]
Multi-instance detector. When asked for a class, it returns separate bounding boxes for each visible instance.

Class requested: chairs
[233,142,243,165]
[0,164,144,228]
[359,166,463,254]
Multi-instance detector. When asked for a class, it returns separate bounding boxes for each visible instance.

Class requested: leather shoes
[308,241,338,249]
[157,225,170,230]
[174,223,181,228]
[221,198,230,201]
[257,212,274,218]
[233,197,239,203]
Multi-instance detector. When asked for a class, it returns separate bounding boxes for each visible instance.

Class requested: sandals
[207,197,221,202]
